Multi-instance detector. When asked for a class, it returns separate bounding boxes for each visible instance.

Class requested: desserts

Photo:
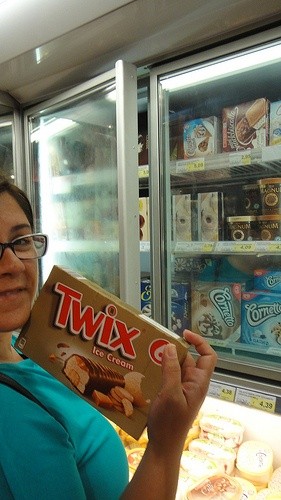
[64,354,148,416]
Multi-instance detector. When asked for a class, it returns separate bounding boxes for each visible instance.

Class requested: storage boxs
[12,62,281,441]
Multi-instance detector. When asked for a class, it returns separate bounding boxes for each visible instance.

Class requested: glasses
[0,232,49,261]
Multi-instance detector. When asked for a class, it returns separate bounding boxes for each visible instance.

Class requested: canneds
[227,177,281,240]
